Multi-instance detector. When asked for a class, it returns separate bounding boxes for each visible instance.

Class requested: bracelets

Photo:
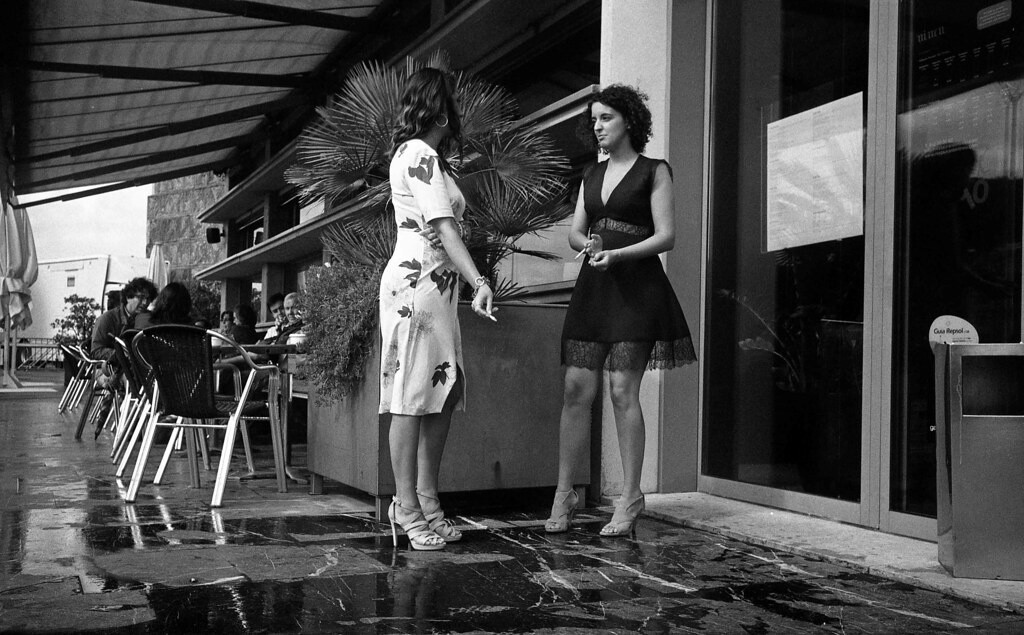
[456,221,471,244]
[470,276,491,299]
[618,249,623,262]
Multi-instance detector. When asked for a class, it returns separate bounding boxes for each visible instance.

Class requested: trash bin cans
[933,340,1024,583]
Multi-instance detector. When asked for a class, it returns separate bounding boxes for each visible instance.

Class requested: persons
[91,277,310,444]
[379,67,499,550]
[545,83,697,536]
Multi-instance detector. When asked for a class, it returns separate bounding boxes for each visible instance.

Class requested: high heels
[388,495,446,550]
[600,493,645,536]
[545,488,579,532]
[416,491,462,541]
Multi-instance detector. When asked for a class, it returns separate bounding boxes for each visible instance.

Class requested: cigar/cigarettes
[574,243,590,260]
[489,315,498,322]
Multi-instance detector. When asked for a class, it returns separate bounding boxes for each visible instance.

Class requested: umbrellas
[0,190,38,389]
[94,357,124,442]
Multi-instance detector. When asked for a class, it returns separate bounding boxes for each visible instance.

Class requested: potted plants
[49,293,105,386]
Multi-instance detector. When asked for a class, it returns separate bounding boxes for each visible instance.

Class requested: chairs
[56,323,288,506]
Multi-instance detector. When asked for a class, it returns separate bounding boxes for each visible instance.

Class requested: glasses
[135,295,152,303]
